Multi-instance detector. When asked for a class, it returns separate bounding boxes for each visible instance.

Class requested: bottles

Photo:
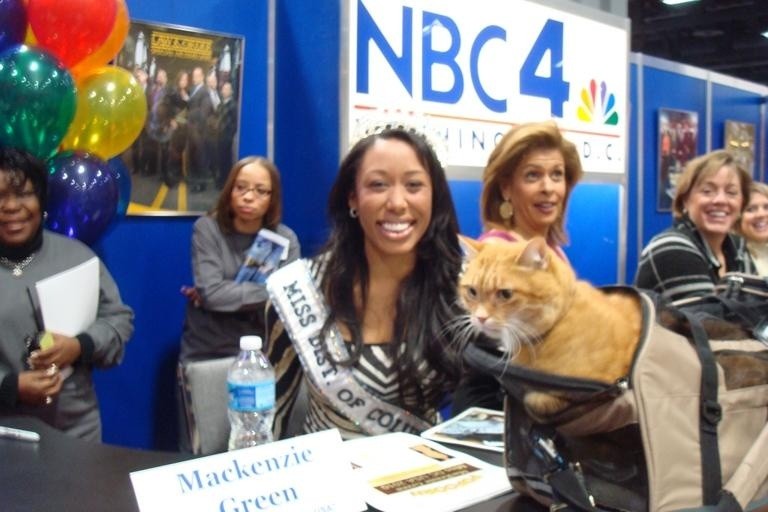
[226,335,277,451]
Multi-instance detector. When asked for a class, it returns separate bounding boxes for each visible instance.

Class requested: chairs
[173,349,310,456]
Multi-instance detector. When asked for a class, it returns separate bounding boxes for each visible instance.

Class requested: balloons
[0,0,148,248]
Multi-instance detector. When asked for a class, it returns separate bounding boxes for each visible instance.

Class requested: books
[420,405,507,455]
[342,431,516,512]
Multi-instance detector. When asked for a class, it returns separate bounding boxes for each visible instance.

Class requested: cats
[453,231,732,436]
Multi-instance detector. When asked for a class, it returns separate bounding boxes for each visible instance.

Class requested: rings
[41,395,54,406]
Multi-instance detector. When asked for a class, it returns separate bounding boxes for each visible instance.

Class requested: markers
[0,426,41,443]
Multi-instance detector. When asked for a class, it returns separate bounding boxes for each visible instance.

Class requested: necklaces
[0,251,38,277]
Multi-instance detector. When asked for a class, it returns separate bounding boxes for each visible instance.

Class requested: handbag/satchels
[461,285,768,512]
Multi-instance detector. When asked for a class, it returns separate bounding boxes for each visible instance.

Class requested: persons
[474,120,583,278]
[732,180,768,283]
[662,113,695,168]
[638,152,757,336]
[0,147,136,443]
[262,120,504,439]
[126,64,238,194]
[179,155,302,454]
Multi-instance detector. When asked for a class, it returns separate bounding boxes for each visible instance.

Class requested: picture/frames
[105,16,245,219]
[655,108,700,212]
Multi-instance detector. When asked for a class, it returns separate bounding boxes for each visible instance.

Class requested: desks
[0,411,528,512]
